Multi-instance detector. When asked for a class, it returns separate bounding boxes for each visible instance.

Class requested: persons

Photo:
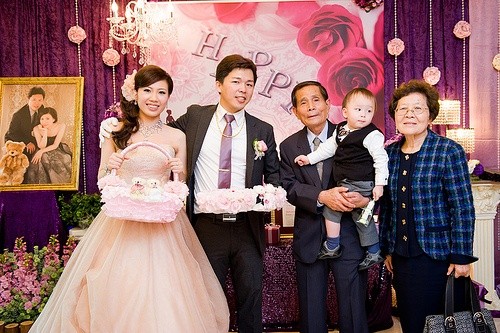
[279,82,390,333]
[383,80,496,333]
[98,54,289,333]
[4,87,72,184]
[27,65,229,333]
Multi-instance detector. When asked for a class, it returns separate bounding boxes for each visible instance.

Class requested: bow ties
[337,126,352,135]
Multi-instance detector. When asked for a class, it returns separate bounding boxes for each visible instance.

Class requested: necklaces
[136,118,163,140]
[215,111,245,137]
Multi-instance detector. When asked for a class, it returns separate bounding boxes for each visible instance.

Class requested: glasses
[394,106,429,115]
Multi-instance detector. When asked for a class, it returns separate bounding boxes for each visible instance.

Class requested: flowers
[468,159,484,175]
[253,141,269,161]
[0,233,79,323]
[121,69,138,102]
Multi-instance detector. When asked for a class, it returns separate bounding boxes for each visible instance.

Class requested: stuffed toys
[0,140,29,185]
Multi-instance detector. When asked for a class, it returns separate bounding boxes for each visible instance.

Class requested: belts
[201,213,244,222]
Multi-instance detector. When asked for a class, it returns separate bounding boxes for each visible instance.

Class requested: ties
[218,115,235,189]
[31,111,38,130]
[313,137,323,183]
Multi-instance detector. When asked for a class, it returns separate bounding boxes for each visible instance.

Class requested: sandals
[317,240,343,259]
[359,249,384,270]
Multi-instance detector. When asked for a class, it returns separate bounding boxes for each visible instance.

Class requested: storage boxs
[265,225,281,244]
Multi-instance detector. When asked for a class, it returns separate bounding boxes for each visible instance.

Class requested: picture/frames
[0,77,85,192]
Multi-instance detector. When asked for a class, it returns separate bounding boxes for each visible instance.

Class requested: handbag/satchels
[97,143,189,223]
[424,270,498,333]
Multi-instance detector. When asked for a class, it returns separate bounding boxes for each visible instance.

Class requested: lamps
[105,0,180,64]
[431,100,475,154]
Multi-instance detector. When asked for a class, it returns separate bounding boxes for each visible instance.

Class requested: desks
[224,239,393,331]
